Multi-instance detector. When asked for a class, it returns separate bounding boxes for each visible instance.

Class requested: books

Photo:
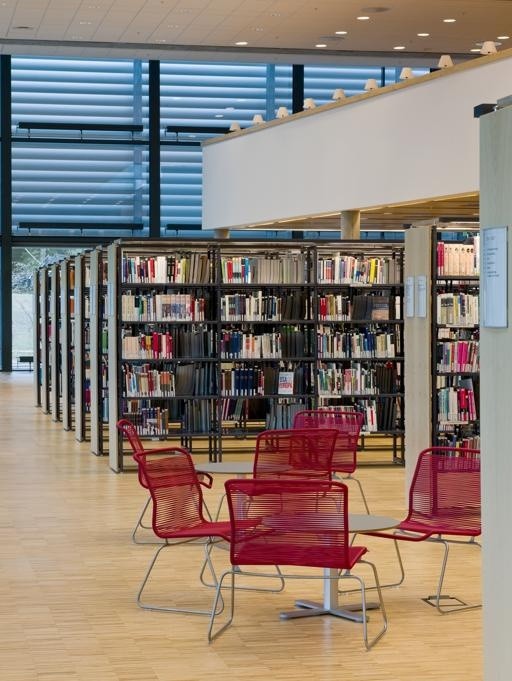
[40,262,110,422]
[124,251,404,436]
[437,242,479,469]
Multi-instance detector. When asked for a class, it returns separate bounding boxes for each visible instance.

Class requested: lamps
[229,40,498,133]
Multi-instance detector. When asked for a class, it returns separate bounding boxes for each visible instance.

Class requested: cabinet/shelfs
[36,240,406,471]
[406,225,481,515]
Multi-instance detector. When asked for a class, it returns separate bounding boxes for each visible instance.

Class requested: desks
[194,462,294,535]
[259,511,400,623]
[16,356,33,371]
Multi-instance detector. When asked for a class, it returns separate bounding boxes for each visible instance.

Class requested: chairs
[247,409,371,515]
[132,446,284,616]
[336,446,481,614]
[205,478,389,649]
[213,428,337,523]
[116,419,215,546]
